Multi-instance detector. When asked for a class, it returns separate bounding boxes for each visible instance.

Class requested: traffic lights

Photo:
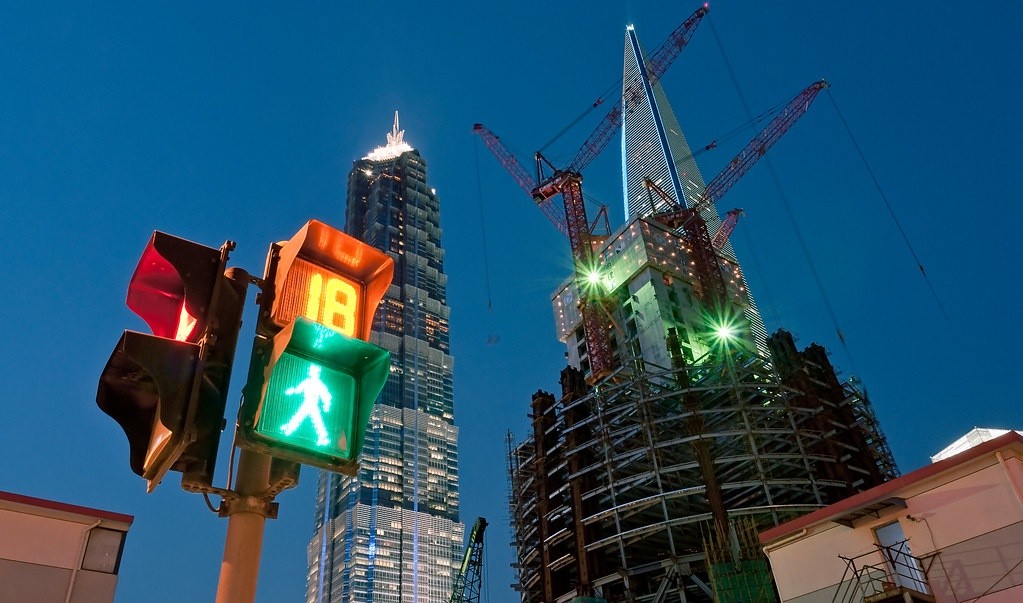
[234,219,395,477]
[95,229,237,496]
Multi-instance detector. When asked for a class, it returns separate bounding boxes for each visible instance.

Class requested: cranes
[472,1,956,396]
[445,516,491,603]
